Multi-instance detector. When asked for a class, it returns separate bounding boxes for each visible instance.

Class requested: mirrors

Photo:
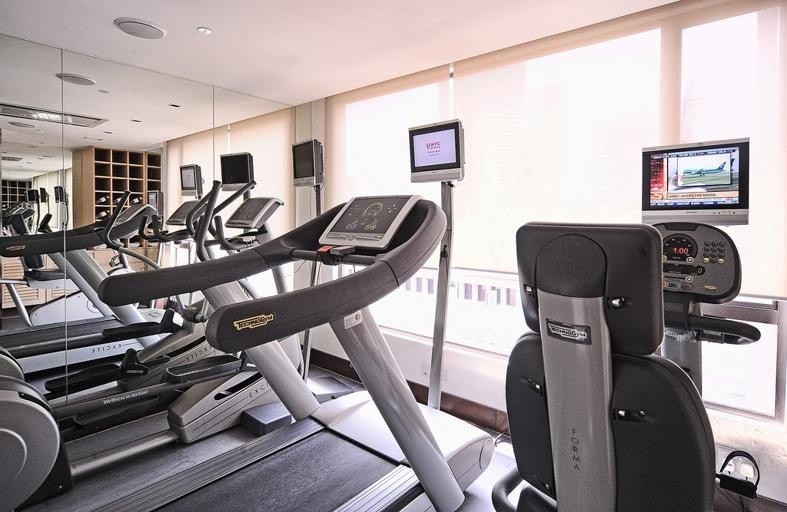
[0,34,297,408]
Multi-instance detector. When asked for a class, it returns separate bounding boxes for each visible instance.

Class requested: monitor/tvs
[27,186,64,203]
[408,118,465,184]
[220,152,254,191]
[115,204,148,224]
[179,164,203,196]
[641,136,750,226]
[318,194,424,251]
[291,137,323,187]
[166,200,207,226]
[224,196,284,229]
[147,189,163,217]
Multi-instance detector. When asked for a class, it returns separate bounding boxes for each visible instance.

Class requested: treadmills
[0,199,183,362]
[95,194,496,512]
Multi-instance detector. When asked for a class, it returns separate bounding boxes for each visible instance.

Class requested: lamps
[56,72,96,86]
[113,16,168,40]
[8,121,38,129]
